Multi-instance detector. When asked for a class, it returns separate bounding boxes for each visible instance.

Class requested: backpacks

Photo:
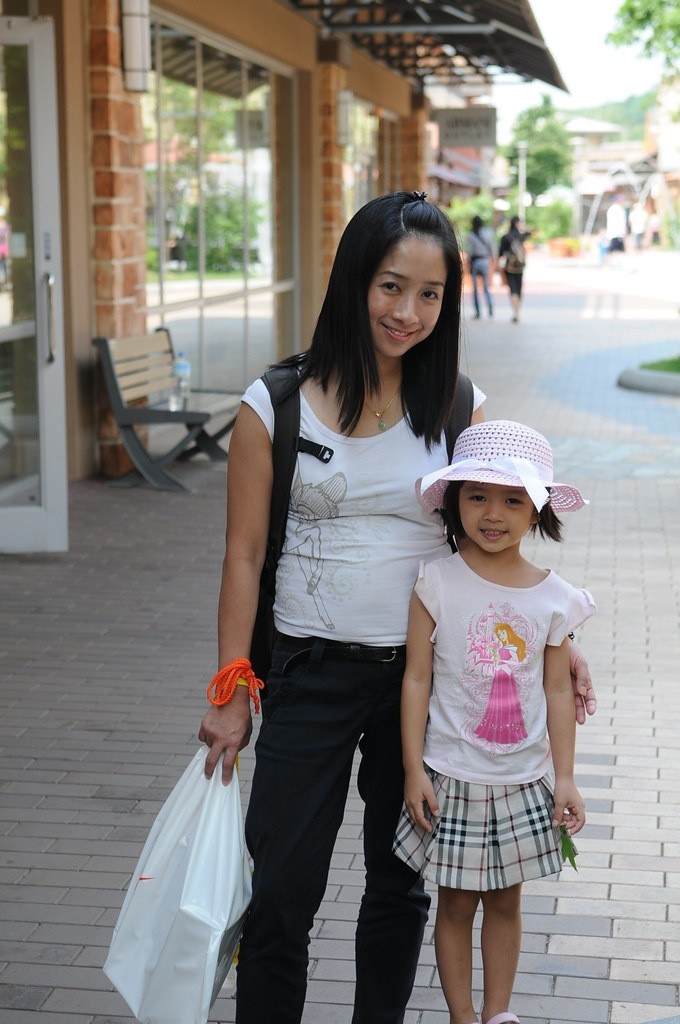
[508,236,526,267]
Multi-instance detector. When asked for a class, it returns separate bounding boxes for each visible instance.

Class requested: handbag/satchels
[99,740,259,1024]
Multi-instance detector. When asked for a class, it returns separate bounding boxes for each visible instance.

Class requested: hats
[415,420,591,514]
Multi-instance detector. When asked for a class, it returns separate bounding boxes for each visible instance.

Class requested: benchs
[90,328,247,493]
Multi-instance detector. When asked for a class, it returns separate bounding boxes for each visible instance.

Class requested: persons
[466,215,497,317]
[199,194,597,1023]
[391,420,586,1024]
[499,217,532,321]
[605,200,658,254]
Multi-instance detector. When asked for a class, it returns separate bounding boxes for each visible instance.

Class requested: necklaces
[363,383,402,432]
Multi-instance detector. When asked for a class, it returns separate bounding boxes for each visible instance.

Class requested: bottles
[169,351,191,412]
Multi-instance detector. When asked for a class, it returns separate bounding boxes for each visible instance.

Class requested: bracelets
[208,658,264,714]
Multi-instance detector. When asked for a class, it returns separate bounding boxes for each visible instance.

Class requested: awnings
[294,0,572,93]
